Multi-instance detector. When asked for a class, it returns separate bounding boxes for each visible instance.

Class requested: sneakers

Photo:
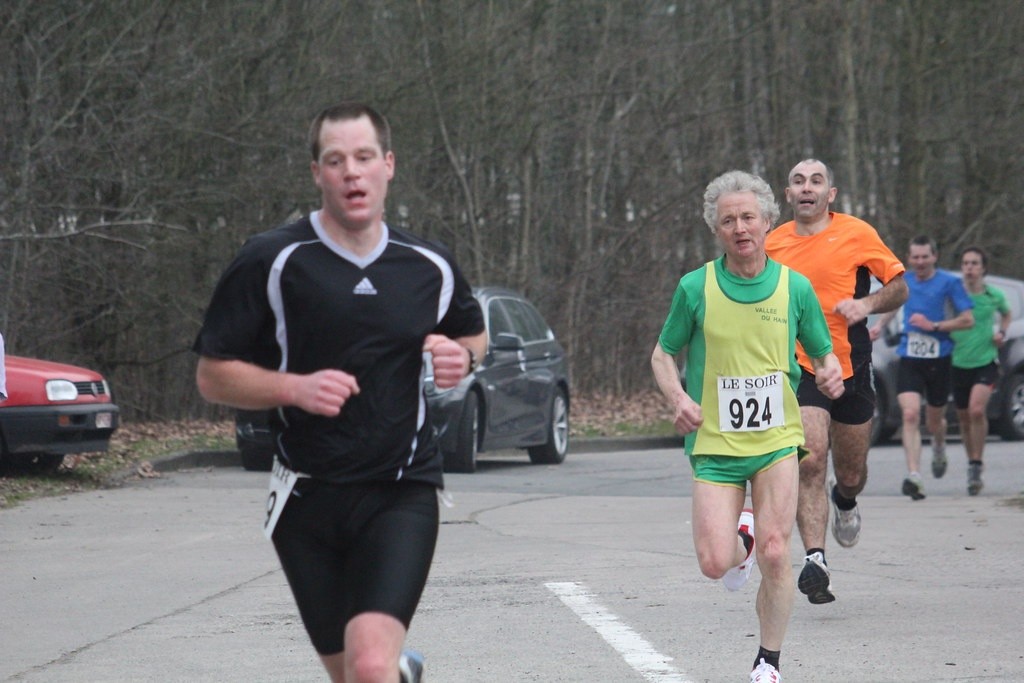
[902,476,928,501]
[929,446,948,479]
[746,657,783,683]
[398,649,428,683]
[796,551,836,605]
[720,507,757,592]
[827,474,863,549]
[965,477,985,497]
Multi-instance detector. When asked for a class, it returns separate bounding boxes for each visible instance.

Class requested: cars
[0,355,122,477]
[864,270,1023,449]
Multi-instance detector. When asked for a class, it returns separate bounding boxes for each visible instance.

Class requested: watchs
[934,321,939,332]
[463,345,476,377]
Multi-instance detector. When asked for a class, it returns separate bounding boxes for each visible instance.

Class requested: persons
[196,102,487,683]
[944,247,1011,494]
[652,171,846,683]
[764,158,908,603]
[869,234,977,502]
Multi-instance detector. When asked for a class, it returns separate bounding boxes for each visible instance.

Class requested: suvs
[233,283,572,472]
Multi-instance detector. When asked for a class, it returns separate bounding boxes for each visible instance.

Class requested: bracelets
[997,329,1006,337]
